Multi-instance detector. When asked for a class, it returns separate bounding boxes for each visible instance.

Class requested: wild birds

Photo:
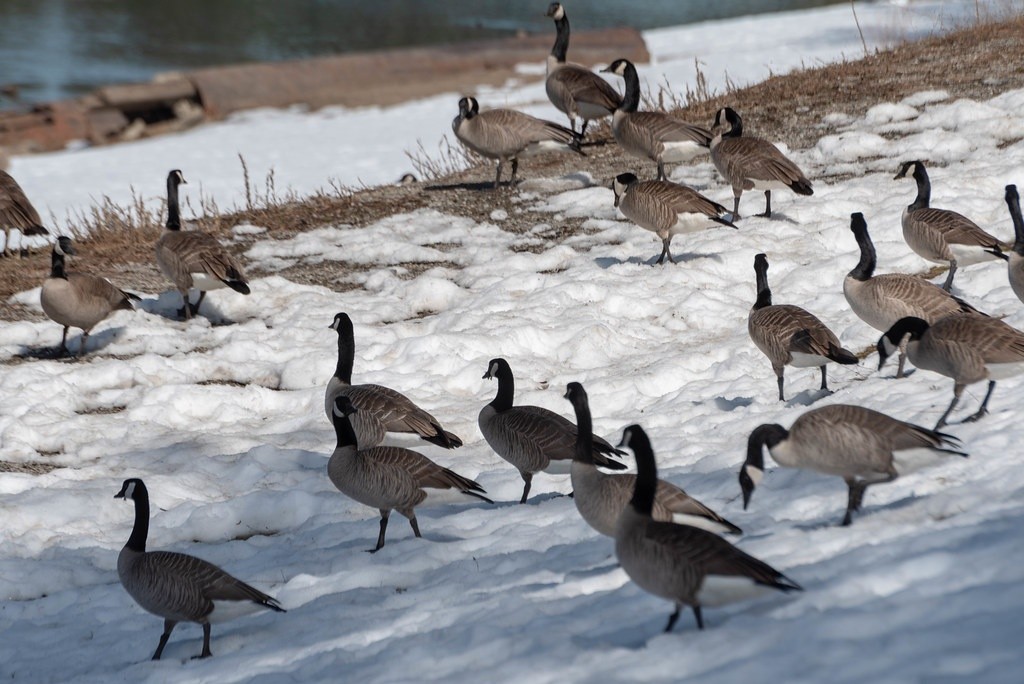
[748,253,859,402]
[41,236,142,356]
[877,314,1024,431]
[156,169,251,320]
[709,106,814,223]
[398,173,417,184]
[599,58,717,181]
[892,159,1024,305]
[843,212,991,334]
[738,403,970,527]
[325,312,463,451]
[328,396,494,554]
[563,382,743,539]
[452,96,584,191]
[616,423,807,633]
[0,169,50,261]
[543,2,624,136]
[612,173,741,265]
[478,358,629,505]
[114,477,288,661]
